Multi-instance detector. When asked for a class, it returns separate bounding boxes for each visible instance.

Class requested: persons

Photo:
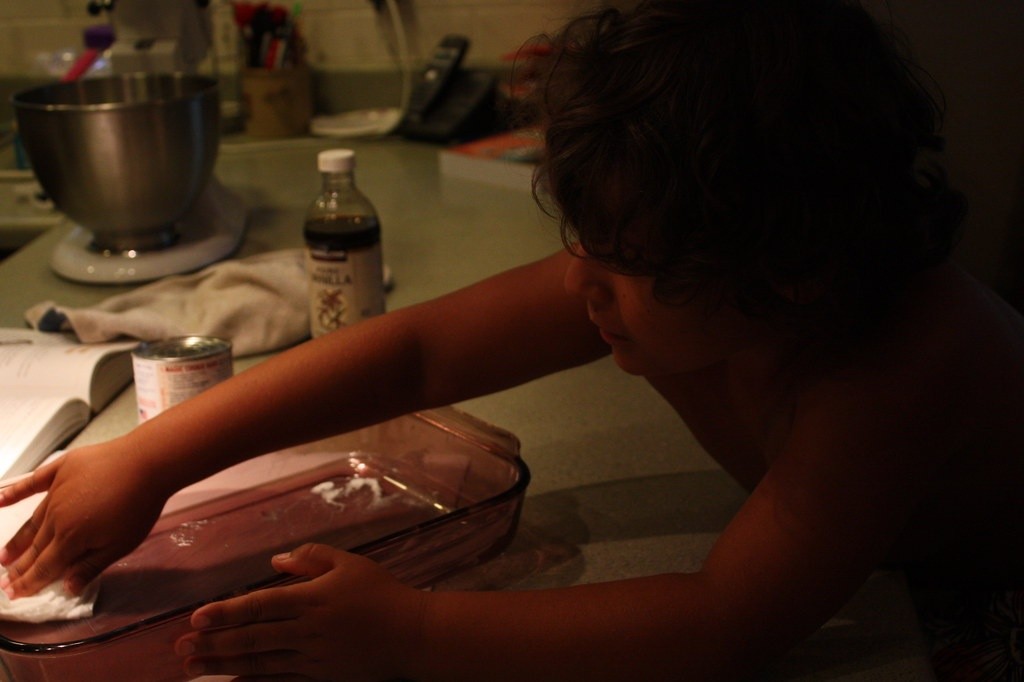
[0,0,1024,682]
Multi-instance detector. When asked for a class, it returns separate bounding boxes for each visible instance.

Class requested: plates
[0,397,531,682]
[47,181,244,282]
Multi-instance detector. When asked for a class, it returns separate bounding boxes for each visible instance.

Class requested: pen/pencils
[238,4,302,71]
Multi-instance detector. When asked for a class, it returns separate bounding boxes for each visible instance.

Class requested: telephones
[398,35,499,148]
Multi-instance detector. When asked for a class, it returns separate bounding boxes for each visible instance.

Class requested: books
[0,325,144,487]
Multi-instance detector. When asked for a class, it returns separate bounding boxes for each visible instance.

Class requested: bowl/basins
[11,73,219,247]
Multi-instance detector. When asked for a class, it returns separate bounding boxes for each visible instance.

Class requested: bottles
[303,150,386,338]
